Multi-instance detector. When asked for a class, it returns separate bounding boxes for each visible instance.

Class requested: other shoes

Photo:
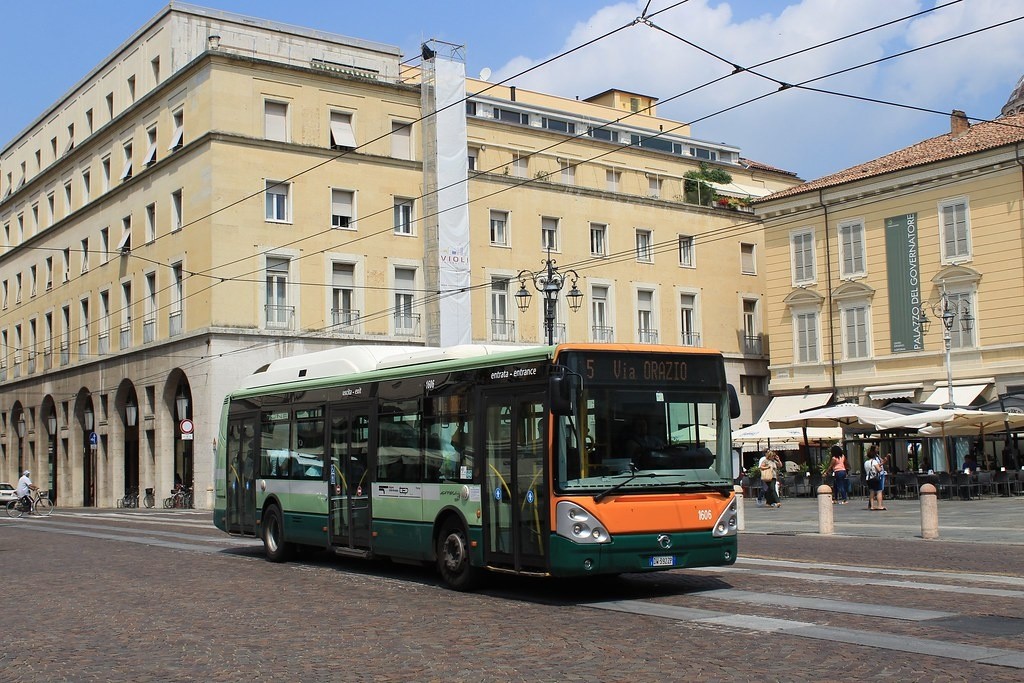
[765,504,772,508]
[771,503,776,507]
[777,502,781,508]
[756,498,762,506]
[832,500,839,504]
[839,501,848,504]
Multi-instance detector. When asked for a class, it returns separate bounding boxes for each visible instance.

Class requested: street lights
[917,279,976,404]
[514,244,584,345]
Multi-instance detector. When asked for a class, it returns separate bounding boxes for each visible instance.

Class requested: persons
[822,446,849,504]
[17,470,36,515]
[950,468,972,500]
[864,445,891,510]
[918,457,931,474]
[630,419,667,450]
[885,463,900,484]
[756,450,782,508]
[428,433,443,477]
[528,419,544,453]
[176,479,182,489]
[963,455,976,471]
[270,449,289,476]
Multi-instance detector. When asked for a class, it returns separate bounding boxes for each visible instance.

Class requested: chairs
[740,470,1024,501]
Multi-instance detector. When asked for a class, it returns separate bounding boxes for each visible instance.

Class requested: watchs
[824,472,827,476]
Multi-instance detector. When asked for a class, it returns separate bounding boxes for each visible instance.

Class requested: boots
[870,509,877,510]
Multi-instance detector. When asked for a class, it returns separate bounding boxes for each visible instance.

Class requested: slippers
[877,507,886,510]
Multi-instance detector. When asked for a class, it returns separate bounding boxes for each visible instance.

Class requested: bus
[213,345,741,591]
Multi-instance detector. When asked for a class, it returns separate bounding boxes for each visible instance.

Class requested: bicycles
[6,488,53,518]
[144,488,155,508]
[164,485,194,509]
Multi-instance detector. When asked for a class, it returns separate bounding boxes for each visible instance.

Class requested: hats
[22,470,30,476]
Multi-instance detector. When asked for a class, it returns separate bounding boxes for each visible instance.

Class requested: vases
[735,204,754,214]
[712,200,734,211]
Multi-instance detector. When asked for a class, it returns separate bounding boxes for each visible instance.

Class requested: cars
[0,482,18,504]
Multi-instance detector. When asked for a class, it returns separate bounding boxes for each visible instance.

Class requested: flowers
[713,197,734,208]
[737,197,753,207]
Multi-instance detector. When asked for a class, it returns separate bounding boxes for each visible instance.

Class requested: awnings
[923,384,988,408]
[757,393,834,422]
[869,389,916,400]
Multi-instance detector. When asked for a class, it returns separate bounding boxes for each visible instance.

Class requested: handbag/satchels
[760,469,773,482]
[867,477,879,489]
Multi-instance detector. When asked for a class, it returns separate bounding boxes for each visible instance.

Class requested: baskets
[39,490,48,498]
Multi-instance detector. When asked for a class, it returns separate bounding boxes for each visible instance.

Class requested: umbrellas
[667,403,906,451]
[875,405,1024,474]
